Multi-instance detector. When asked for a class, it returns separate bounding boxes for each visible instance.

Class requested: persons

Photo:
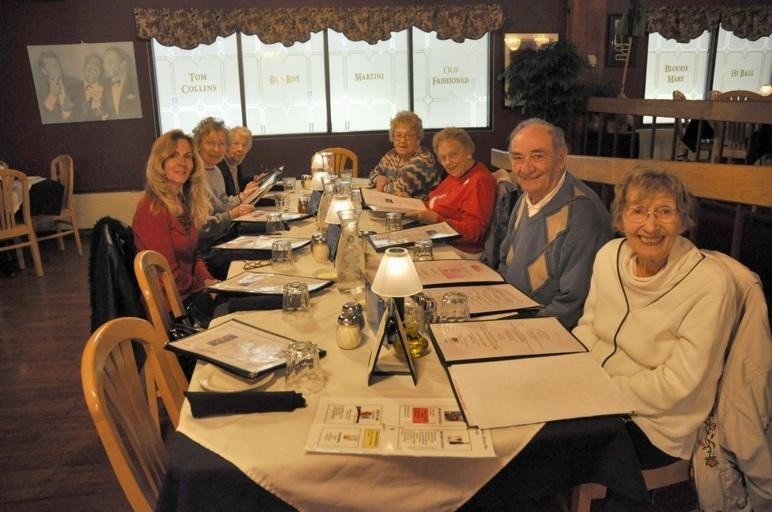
[36,47,144,124]
[191,117,259,280]
[399,127,496,261]
[498,118,612,329]
[132,129,225,320]
[569,165,736,470]
[217,125,268,196]
[367,111,442,201]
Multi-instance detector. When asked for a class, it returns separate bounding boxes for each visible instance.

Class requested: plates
[198,362,277,393]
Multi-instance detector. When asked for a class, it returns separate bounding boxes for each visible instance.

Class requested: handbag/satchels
[166,302,211,339]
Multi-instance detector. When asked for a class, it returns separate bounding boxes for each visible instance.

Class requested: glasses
[393,132,417,141]
[358,230,376,237]
[243,259,271,270]
[622,205,678,225]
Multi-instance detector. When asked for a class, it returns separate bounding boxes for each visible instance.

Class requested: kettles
[321,152,335,175]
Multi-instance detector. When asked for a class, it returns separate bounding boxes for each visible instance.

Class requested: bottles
[311,232,329,263]
[298,175,312,213]
[413,293,438,323]
[336,301,365,349]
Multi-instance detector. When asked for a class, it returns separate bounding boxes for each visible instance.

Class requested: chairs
[311,148,358,179]
[133,248,193,400]
[87,215,151,374]
[80,316,189,512]
[555,249,772,496]
[491,166,514,271]
[0,154,84,277]
[671,90,772,261]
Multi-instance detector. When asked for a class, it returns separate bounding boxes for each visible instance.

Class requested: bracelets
[239,192,246,200]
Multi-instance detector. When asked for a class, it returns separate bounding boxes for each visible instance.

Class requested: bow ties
[110,80,121,87]
[86,83,93,89]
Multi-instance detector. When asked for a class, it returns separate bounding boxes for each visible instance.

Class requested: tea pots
[318,176,339,232]
[335,211,367,294]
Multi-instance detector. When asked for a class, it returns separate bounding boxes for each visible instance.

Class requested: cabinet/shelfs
[568,121,640,213]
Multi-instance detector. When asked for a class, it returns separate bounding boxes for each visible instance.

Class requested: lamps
[311,153,324,169]
[324,194,355,229]
[309,172,331,192]
[368,246,423,345]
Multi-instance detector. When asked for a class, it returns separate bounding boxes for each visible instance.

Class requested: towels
[236,222,289,235]
[255,197,276,207]
[227,295,282,313]
[269,183,284,192]
[181,387,307,419]
[213,247,273,261]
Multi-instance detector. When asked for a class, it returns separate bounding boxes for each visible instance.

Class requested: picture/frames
[499,30,564,110]
[605,13,638,68]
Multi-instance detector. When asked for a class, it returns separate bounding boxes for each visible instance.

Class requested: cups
[265,175,296,235]
[338,172,361,206]
[271,240,295,272]
[440,293,470,323]
[285,341,326,394]
[282,283,313,325]
[413,240,433,261]
[385,212,403,233]
